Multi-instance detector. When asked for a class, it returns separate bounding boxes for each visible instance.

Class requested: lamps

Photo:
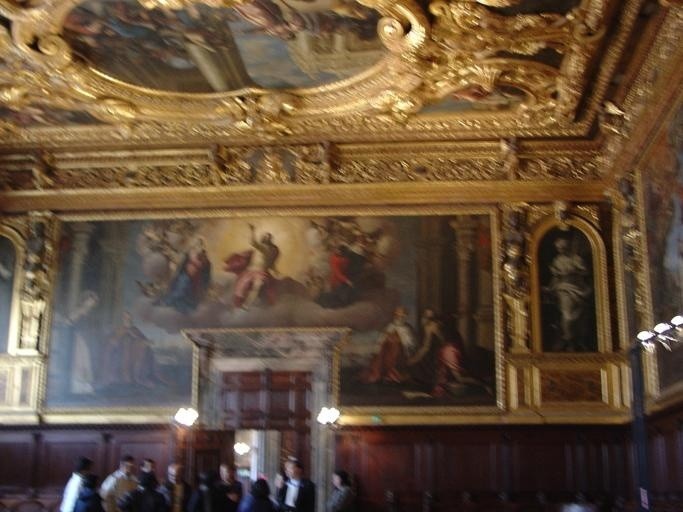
[636,315,683,353]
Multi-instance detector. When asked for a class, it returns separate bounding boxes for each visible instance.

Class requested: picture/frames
[632,84,683,416]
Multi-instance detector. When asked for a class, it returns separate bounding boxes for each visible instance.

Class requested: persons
[57,456,98,512]
[223,223,280,309]
[97,455,317,512]
[401,308,495,401]
[99,312,162,390]
[369,303,434,384]
[326,224,378,296]
[157,242,211,310]
[325,469,356,512]
[537,235,595,342]
